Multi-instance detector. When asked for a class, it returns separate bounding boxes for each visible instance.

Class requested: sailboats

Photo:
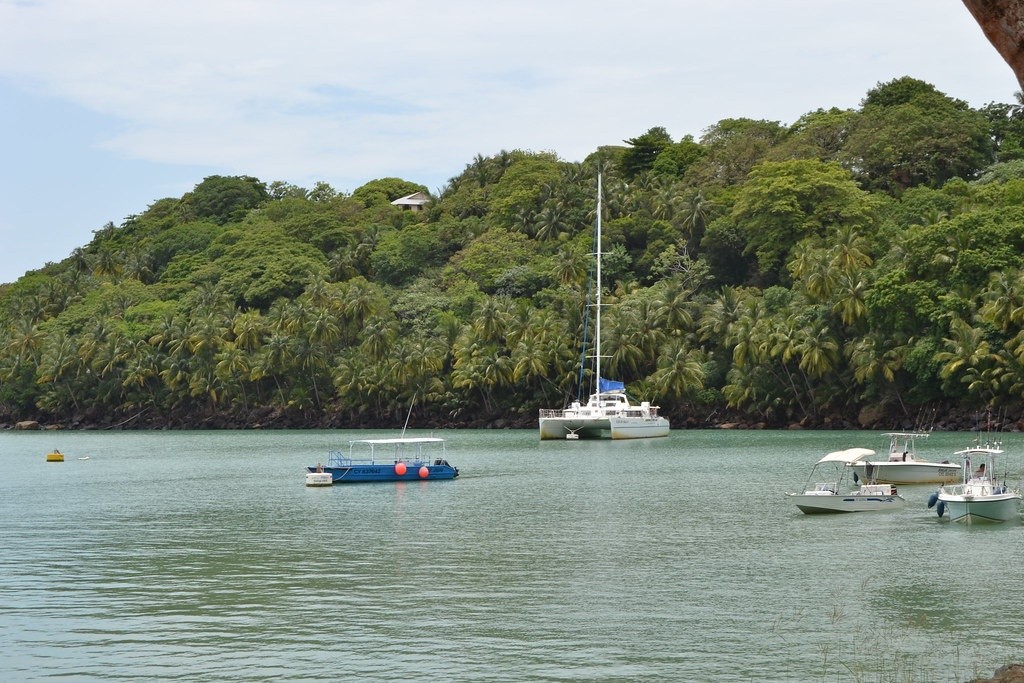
[540,173,669,440]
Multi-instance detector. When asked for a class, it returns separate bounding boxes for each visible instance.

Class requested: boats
[785,448,905,514]
[47,450,64,461]
[845,397,961,485]
[937,406,1022,526]
[306,390,460,486]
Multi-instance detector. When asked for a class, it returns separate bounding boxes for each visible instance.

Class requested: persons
[903,452,908,461]
[973,464,986,478]
